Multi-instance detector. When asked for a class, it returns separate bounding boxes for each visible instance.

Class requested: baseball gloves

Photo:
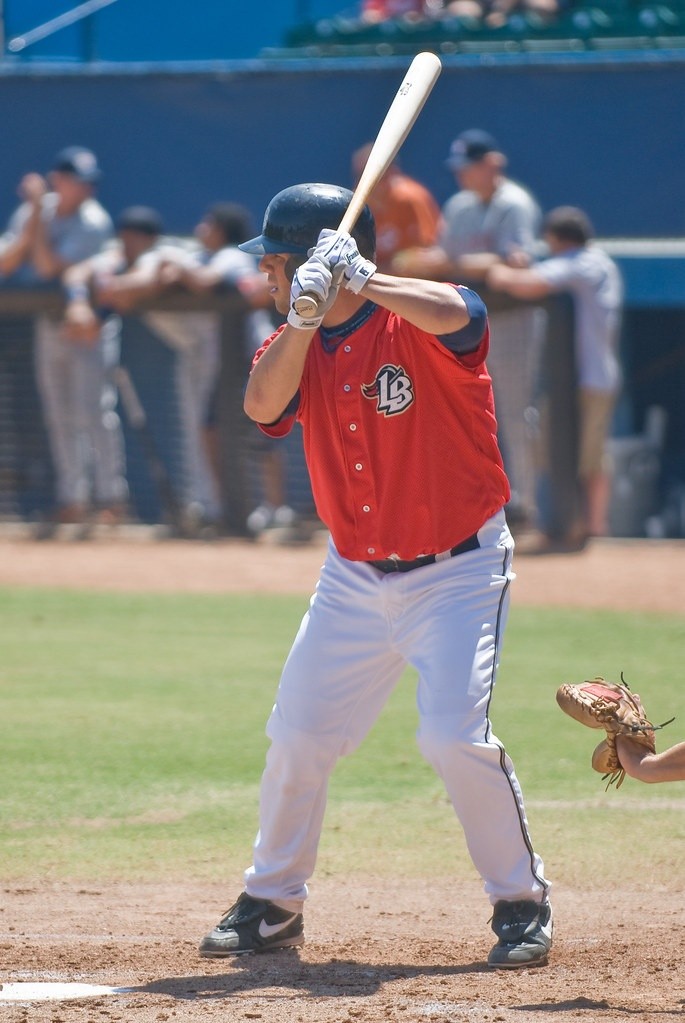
[555,675,657,793]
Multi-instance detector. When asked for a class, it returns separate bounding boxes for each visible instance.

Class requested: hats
[51,148,99,182]
[443,129,496,171]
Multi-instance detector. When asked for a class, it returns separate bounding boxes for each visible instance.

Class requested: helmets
[239,182,376,256]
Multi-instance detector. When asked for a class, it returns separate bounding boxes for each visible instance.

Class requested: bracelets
[63,282,90,299]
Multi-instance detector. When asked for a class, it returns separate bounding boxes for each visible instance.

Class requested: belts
[368,533,481,574]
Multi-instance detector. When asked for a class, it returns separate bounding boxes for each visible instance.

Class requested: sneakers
[485,900,554,966]
[198,892,306,956]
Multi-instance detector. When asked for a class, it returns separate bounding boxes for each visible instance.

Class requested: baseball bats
[294,50,443,320]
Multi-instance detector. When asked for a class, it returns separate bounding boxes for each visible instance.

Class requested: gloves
[286,254,348,330]
[307,228,377,295]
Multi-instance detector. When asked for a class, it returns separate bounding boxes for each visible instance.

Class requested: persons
[60,206,223,536]
[0,145,129,525]
[555,676,684,784]
[350,141,443,266]
[162,202,276,541]
[390,130,548,552]
[196,183,554,967]
[362,0,559,25]
[483,204,623,545]
